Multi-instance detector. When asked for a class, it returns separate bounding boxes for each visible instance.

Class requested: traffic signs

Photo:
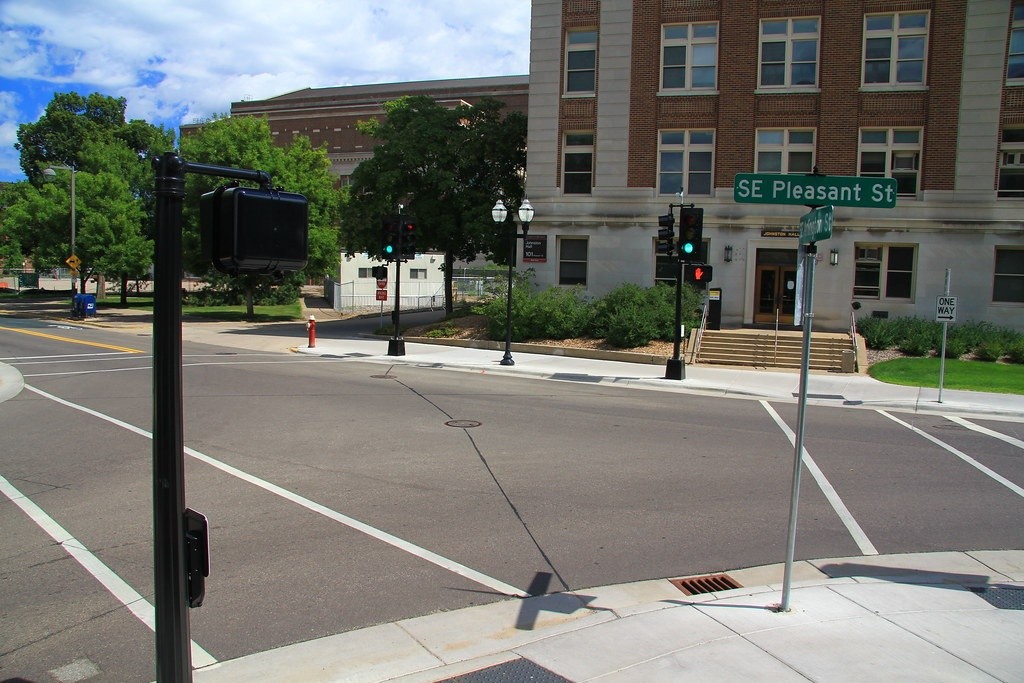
[936,296,957,323]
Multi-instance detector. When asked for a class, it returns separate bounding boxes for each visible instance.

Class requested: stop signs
[377,277,388,287]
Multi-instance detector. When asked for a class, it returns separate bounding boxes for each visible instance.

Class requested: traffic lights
[402,223,414,255]
[656,215,675,258]
[383,225,396,259]
[680,208,703,260]
[692,264,713,284]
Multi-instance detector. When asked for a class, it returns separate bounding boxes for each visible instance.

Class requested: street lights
[43,165,78,315]
[491,191,535,366]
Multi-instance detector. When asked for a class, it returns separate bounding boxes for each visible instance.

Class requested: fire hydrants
[306,313,316,349]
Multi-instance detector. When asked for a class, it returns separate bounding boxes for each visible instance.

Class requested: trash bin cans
[74,293,97,317]
